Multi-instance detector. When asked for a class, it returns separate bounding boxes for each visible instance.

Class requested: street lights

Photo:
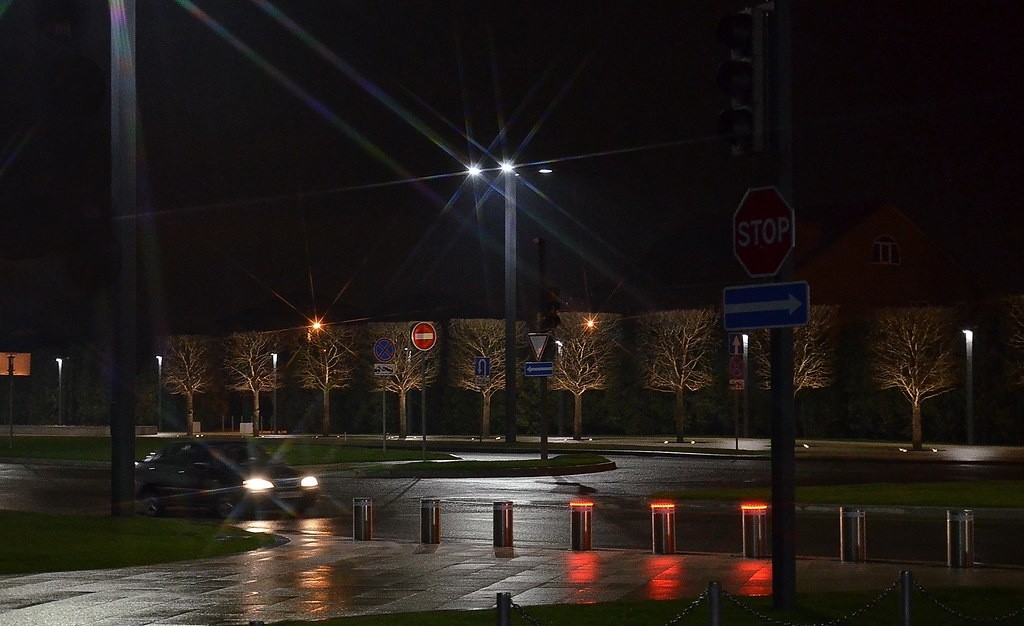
[268,353,281,433]
[465,153,524,446]
[154,354,164,431]
[53,357,66,425]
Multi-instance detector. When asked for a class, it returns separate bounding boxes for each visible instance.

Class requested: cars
[134,438,321,518]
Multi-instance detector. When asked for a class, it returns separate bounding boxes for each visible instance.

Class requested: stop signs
[411,322,437,353]
[732,186,795,279]
